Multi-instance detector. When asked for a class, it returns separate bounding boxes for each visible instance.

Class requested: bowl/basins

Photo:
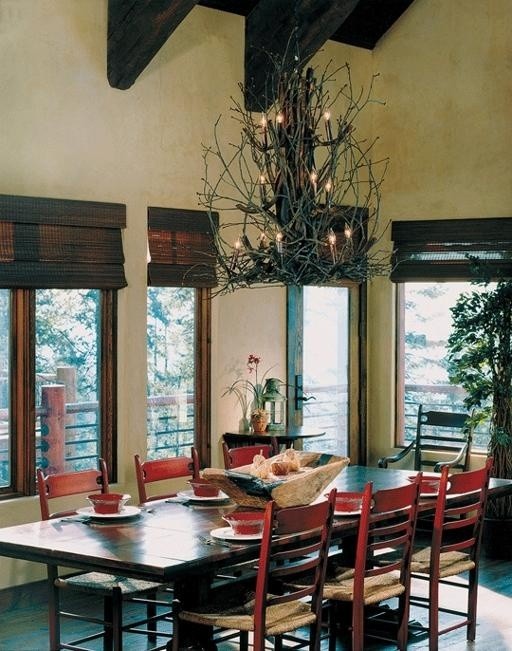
[221,510,268,533]
[185,478,223,497]
[324,493,367,512]
[86,494,131,514]
[407,476,444,491]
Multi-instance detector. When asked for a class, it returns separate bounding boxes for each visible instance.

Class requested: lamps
[180,0,413,300]
[262,379,285,431]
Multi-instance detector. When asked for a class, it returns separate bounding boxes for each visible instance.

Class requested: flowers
[230,353,284,410]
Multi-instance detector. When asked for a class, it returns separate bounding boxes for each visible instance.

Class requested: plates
[177,490,231,505]
[210,528,279,542]
[76,506,142,521]
[400,481,451,497]
[310,501,376,516]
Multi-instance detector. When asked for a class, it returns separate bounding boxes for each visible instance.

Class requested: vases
[251,409,269,432]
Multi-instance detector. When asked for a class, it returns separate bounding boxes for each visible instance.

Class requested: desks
[0,465,512,651]
[224,426,326,449]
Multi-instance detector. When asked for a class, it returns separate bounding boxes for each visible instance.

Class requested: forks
[198,535,232,548]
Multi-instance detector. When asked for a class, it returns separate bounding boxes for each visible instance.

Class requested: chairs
[222,436,279,470]
[378,404,476,542]
[281,472,423,651]
[135,447,250,650]
[37,457,162,651]
[180,488,338,651]
[397,457,493,651]
